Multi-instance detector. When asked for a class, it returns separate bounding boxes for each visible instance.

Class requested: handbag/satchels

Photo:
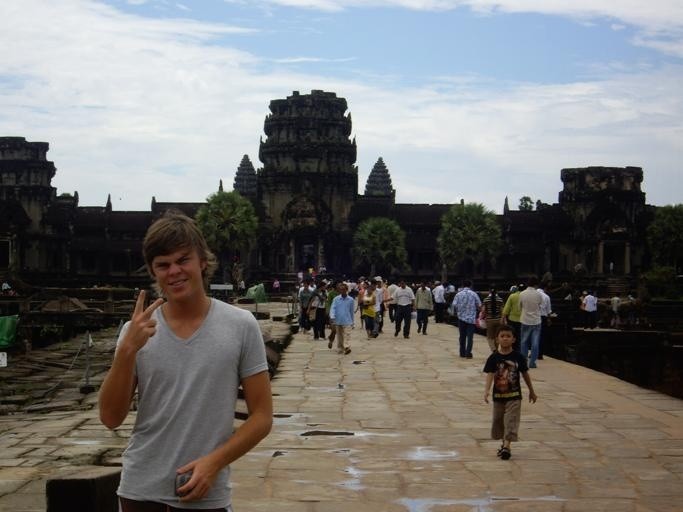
[309,309,316,321]
[477,318,487,329]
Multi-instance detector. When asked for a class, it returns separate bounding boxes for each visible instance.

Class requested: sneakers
[394,327,427,338]
[328,343,351,354]
[461,352,472,357]
[497,445,511,460]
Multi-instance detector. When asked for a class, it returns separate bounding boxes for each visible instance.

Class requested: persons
[480,267,598,368]
[274,278,280,294]
[296,264,456,354]
[98,214,273,512]
[451,279,482,359]
[2,280,11,292]
[484,325,537,459]
[609,291,638,330]
